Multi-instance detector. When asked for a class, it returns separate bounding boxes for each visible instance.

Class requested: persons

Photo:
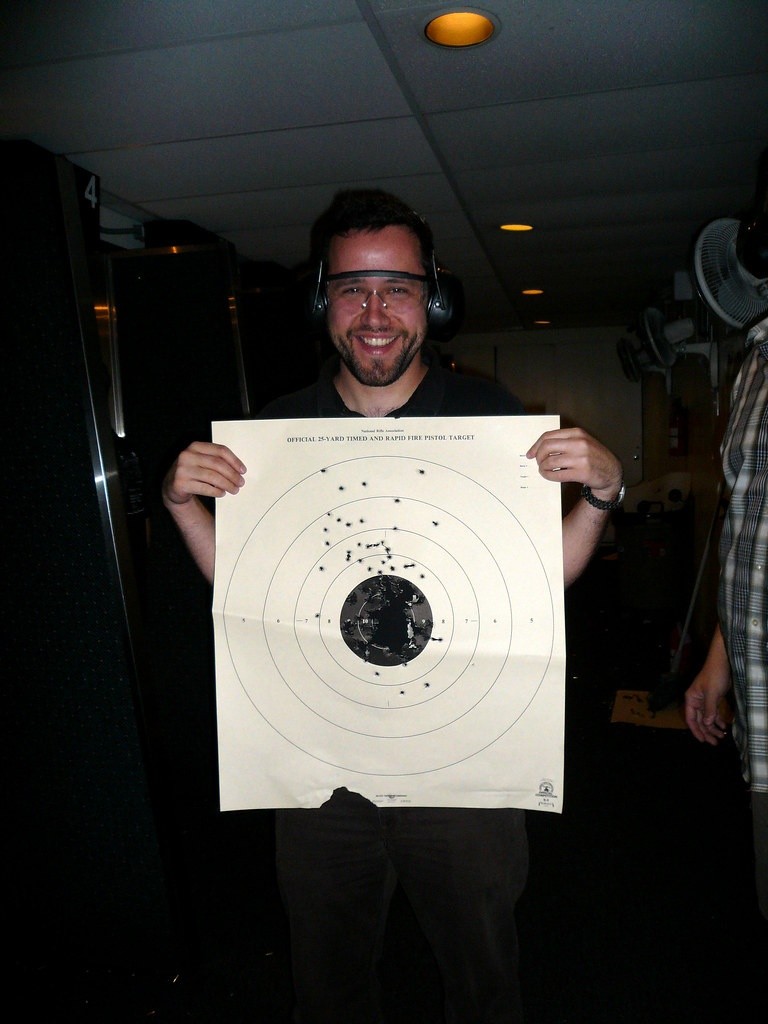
[684,316,768,916]
[164,187,629,1024]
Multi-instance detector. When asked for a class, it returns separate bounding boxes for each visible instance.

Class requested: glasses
[318,269,431,314]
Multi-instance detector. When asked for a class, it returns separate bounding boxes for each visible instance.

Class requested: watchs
[581,482,627,510]
